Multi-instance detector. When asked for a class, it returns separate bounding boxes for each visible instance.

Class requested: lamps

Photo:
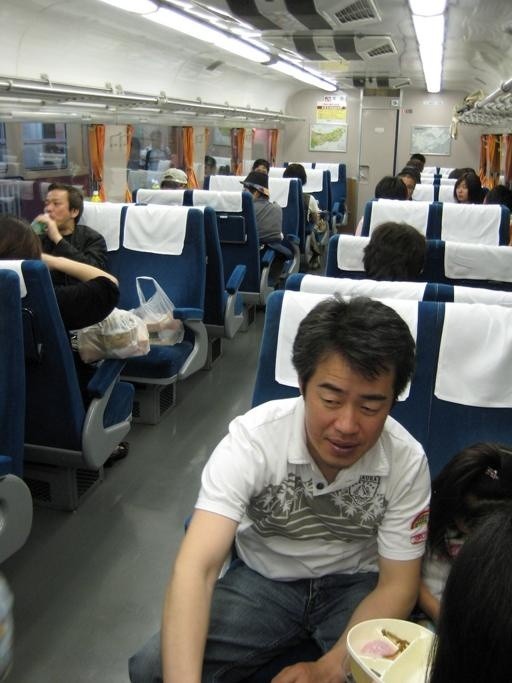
[406,0,447,95]
[100,1,339,93]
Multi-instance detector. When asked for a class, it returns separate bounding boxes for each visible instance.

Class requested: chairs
[1,160,347,680]
[128,165,512,682]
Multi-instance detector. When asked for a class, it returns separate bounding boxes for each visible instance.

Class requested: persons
[33,179,111,292]
[360,218,427,281]
[348,174,406,236]
[245,159,271,175]
[282,160,324,267]
[0,212,134,478]
[158,166,191,190]
[234,170,284,262]
[123,288,431,683]
[481,183,512,217]
[418,436,512,681]
[195,155,219,174]
[396,150,485,203]
[141,128,175,169]
[424,502,511,683]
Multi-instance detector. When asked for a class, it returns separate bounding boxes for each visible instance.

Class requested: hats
[238,169,272,199]
[160,165,190,184]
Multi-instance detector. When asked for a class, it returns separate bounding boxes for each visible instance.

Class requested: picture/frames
[309,122,348,153]
[409,124,452,156]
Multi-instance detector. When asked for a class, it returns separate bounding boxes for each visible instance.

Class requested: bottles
[91,190,101,203]
[30,213,51,237]
